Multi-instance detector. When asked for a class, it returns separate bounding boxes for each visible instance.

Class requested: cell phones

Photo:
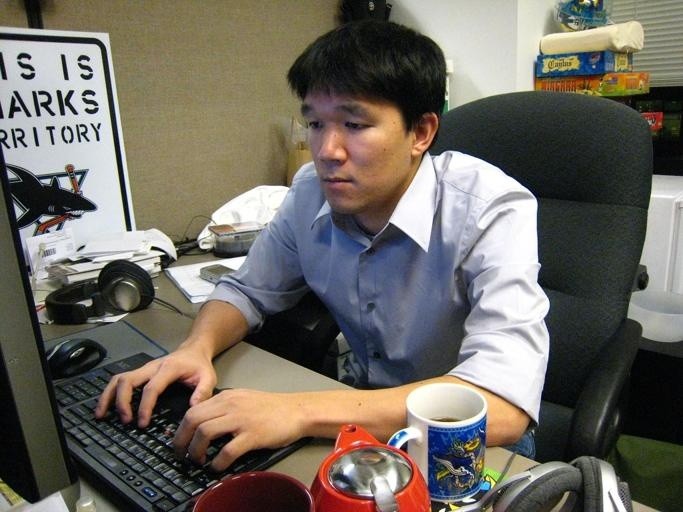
[196,262,236,285]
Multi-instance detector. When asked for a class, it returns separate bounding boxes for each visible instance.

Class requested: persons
[95,19,551,471]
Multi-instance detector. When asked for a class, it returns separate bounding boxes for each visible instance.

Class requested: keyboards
[43,351,314,512]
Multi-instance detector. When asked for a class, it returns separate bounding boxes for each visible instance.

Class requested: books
[44,229,177,287]
[164,255,248,304]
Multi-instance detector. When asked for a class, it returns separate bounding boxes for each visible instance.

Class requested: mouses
[41,336,108,379]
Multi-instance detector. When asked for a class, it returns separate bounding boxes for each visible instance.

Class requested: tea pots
[311,419,433,511]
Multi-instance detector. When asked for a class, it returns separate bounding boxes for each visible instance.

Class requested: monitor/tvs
[0,149,79,506]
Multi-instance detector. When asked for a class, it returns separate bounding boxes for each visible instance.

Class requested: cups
[387,382,488,504]
[191,469,317,512]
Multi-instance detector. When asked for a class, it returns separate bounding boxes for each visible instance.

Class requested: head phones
[43,259,155,327]
[447,454,634,512]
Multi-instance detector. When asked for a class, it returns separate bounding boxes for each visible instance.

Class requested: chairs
[250,92,653,462]
